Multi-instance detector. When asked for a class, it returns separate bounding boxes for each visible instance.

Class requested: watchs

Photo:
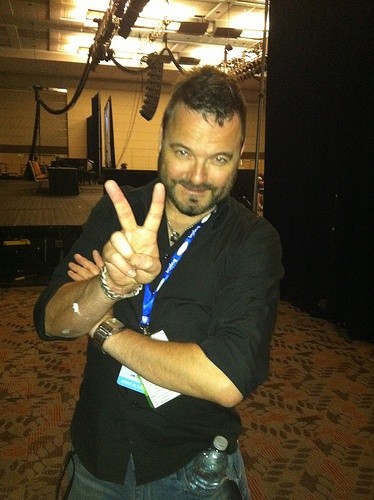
[92,317,127,355]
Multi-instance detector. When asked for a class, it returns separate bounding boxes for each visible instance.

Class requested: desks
[46,167,80,196]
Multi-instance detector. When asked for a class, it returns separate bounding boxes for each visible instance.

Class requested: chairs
[29,160,49,194]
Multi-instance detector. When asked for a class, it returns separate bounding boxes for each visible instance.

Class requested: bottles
[188,436,229,493]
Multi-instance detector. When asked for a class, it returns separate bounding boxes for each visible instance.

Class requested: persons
[34,65,283,499]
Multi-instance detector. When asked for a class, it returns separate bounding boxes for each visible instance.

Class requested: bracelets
[99,263,143,301]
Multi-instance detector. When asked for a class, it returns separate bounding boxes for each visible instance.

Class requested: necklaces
[168,219,198,244]
